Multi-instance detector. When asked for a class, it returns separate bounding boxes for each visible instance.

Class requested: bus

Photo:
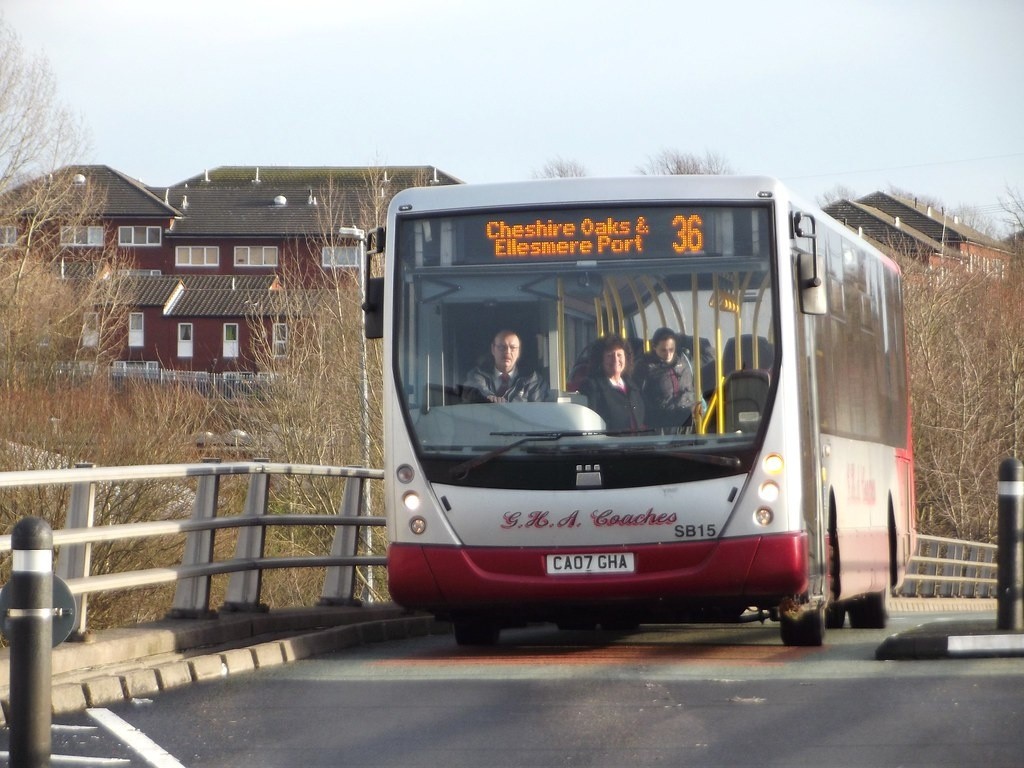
[360,175,918,647]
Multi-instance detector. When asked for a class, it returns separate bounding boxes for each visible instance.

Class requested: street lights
[338,227,373,604]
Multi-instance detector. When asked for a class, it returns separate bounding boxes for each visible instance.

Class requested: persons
[631,326,707,436]
[459,326,546,405]
[581,333,647,434]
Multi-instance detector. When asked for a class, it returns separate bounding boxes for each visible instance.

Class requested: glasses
[494,343,519,353]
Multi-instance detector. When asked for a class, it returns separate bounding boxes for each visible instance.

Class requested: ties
[499,372,510,397]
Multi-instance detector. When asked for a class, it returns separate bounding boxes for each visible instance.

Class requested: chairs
[569,332,774,412]
